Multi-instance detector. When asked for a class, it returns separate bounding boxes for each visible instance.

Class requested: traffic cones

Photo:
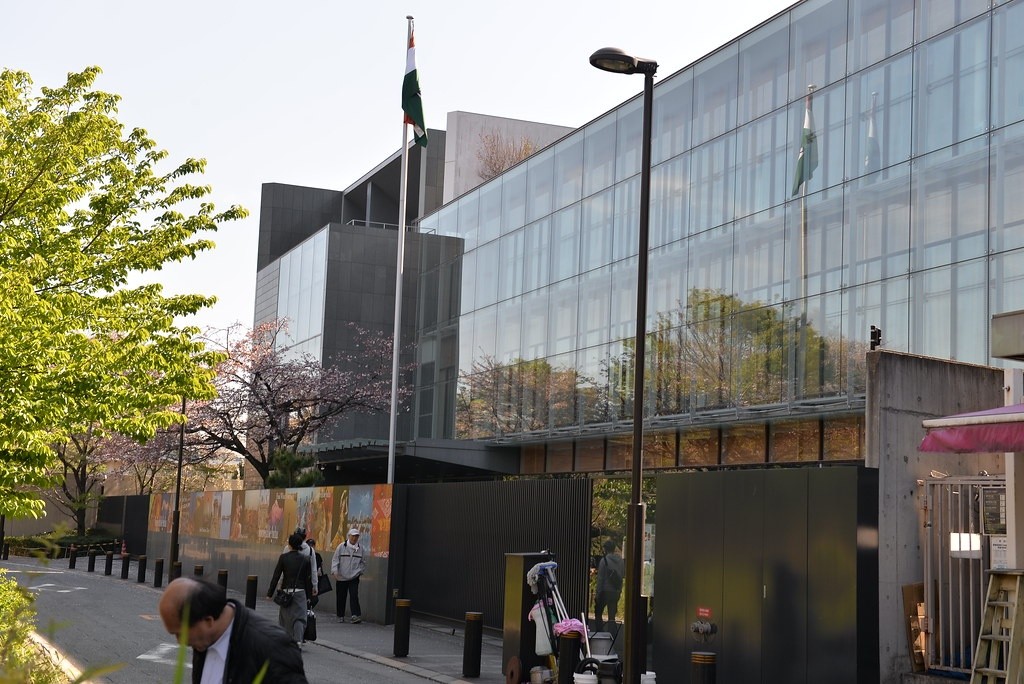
[120,539,127,559]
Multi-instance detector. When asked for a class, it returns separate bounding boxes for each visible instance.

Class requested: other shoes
[336,617,344,623]
[350,615,361,624]
[297,641,301,649]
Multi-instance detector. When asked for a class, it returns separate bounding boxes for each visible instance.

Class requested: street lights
[589,48,661,684]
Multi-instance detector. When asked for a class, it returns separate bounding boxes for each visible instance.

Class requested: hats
[348,528,360,536]
[293,526,306,536]
[306,538,316,545]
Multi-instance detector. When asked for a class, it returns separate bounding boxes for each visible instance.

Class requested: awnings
[917,401,1024,453]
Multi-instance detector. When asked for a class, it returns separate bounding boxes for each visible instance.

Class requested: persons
[306,538,323,607]
[594,540,624,639]
[331,529,366,624]
[265,534,312,649]
[281,528,319,645]
[160,575,309,684]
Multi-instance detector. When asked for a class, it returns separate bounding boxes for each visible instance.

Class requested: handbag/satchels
[273,589,294,609]
[302,606,317,640]
[607,567,623,589]
[317,564,332,595]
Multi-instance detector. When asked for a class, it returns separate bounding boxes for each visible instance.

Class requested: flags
[791,97,820,199]
[862,131,884,212]
[400,22,428,148]
